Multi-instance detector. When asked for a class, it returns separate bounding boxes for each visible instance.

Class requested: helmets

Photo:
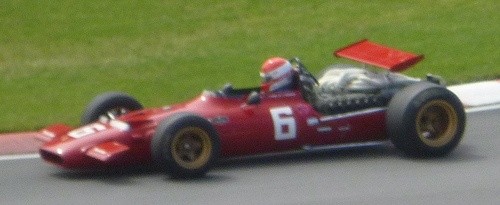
[260,58,293,94]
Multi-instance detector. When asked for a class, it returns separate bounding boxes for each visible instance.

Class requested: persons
[241,56,302,102]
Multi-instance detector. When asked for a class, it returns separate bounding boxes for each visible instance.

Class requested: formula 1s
[37,38,467,180]
